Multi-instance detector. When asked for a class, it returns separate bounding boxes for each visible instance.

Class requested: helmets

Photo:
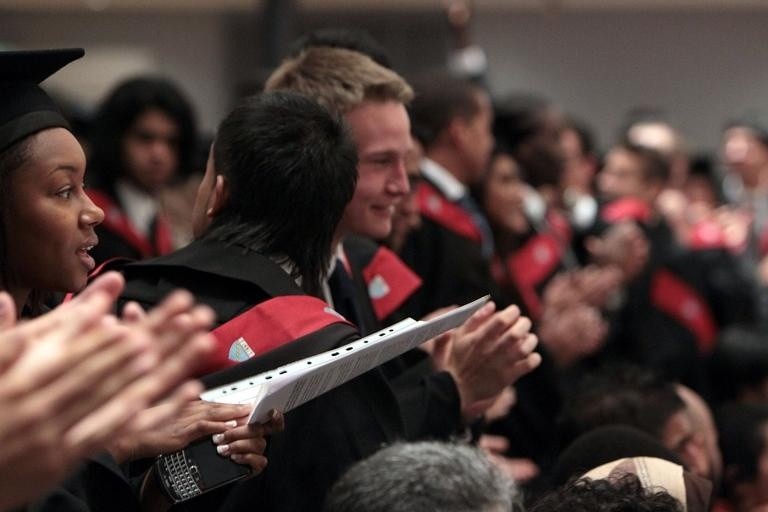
[0,47,85,151]
